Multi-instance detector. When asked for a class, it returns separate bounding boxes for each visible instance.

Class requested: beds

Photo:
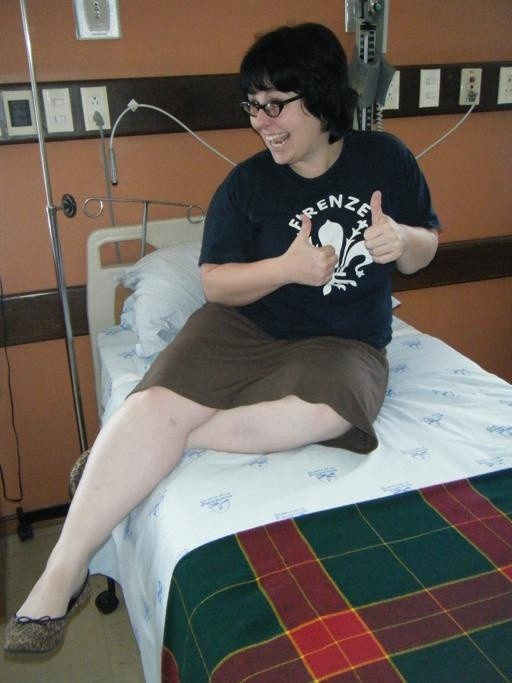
[83,208,512,683]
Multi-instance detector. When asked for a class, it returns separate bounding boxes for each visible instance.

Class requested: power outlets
[79,85,111,131]
[73,0,120,42]
[459,67,482,105]
[497,67,512,105]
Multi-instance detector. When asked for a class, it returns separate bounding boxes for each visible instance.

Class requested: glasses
[239,93,307,118]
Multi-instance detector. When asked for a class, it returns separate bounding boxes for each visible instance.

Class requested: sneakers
[3,568,92,661]
[69,447,91,499]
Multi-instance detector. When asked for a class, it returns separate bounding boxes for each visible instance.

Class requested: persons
[3,19,442,663]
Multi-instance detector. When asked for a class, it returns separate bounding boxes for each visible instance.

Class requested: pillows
[113,238,213,358]
